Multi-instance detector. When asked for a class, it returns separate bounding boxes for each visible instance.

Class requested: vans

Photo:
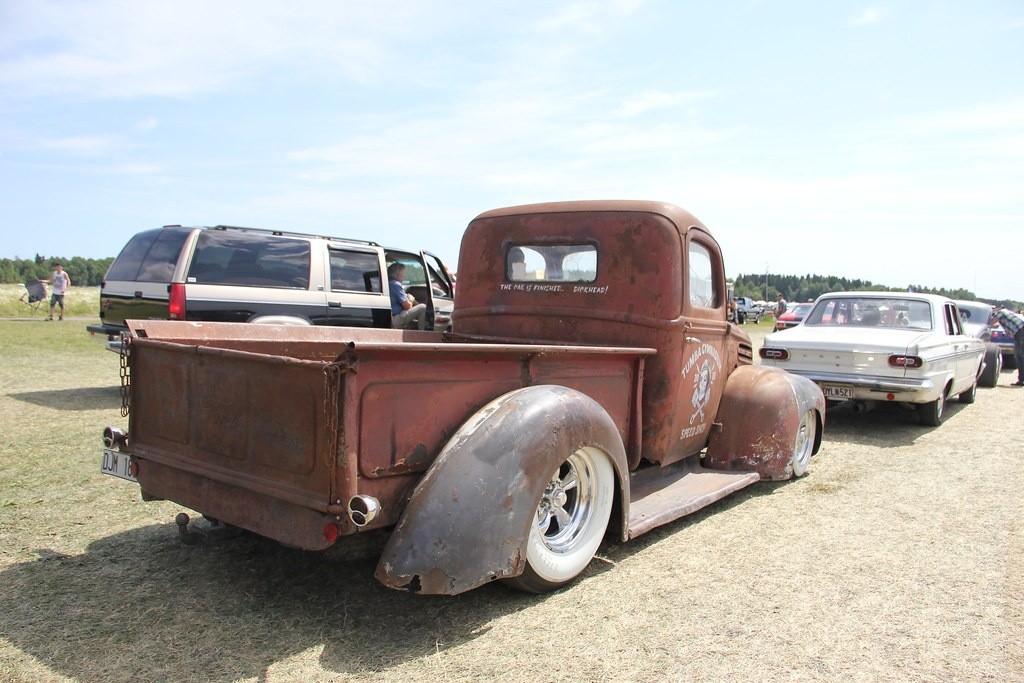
[85,224,458,359]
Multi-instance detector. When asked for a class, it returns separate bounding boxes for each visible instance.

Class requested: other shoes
[57,315,63,321]
[45,315,54,321]
[1010,382,1024,386]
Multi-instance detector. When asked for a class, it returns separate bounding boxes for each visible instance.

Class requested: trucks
[99,199,825,595]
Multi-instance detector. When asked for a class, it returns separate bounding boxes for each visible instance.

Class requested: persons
[772,293,786,331]
[990,306,1024,386]
[728,297,739,325]
[39,261,71,322]
[388,263,426,331]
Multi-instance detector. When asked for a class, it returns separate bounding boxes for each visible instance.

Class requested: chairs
[405,286,444,302]
[860,304,880,326]
[508,248,524,278]
[19,280,49,316]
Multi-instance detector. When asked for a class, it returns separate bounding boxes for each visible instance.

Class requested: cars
[952,300,1017,390]
[760,291,988,426]
[735,295,853,335]
